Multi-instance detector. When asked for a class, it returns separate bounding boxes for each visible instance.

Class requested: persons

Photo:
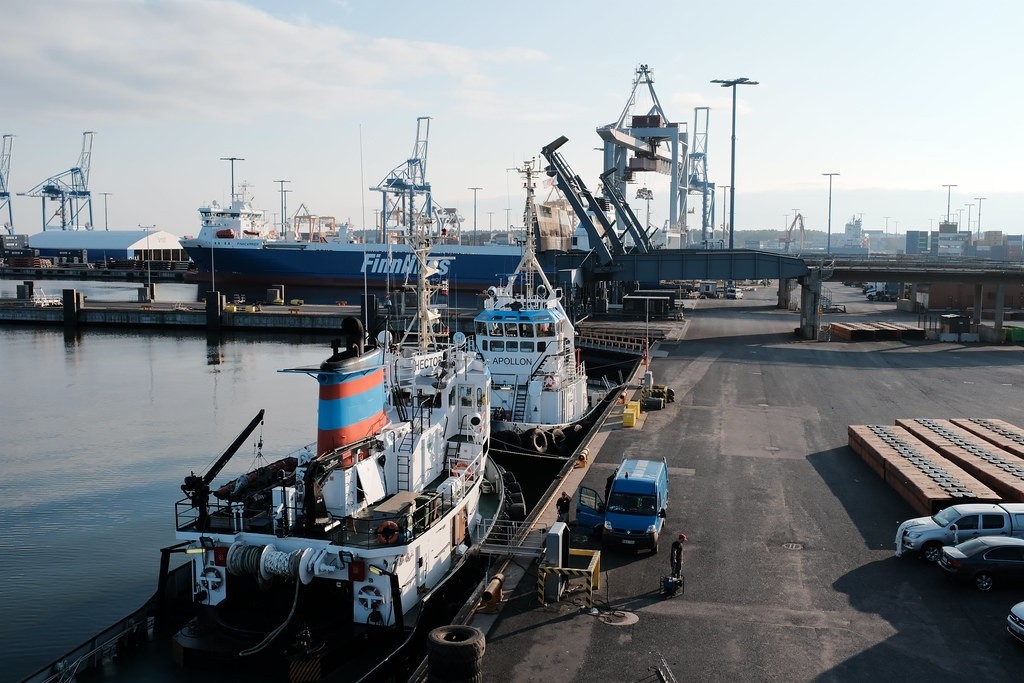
[556,491,572,523]
[669,534,686,579]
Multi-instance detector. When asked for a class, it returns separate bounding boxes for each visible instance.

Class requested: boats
[19,155,526,683]
[455,160,624,456]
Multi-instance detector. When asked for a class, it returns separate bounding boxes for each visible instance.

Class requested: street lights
[941,215,947,220]
[791,209,800,246]
[219,157,245,200]
[928,219,936,231]
[822,172,840,253]
[277,189,293,240]
[965,204,975,231]
[487,212,494,233]
[950,214,957,222]
[709,73,759,284]
[503,209,512,230]
[974,197,987,240]
[273,179,292,235]
[783,215,790,236]
[957,209,965,231]
[467,187,484,246]
[883,216,891,238]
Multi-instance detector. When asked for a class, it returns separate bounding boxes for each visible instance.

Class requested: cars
[1005,600,1024,643]
[937,535,1024,593]
[896,503,1024,566]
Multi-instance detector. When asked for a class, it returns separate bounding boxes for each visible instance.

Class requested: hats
[679,533,688,542]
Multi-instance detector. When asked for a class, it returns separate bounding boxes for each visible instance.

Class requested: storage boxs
[628,401,640,418]
[939,333,958,342]
[623,409,636,427]
[961,333,979,342]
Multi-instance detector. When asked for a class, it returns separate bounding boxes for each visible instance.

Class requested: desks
[374,491,420,522]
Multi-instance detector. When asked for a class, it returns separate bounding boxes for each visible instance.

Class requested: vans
[575,458,669,553]
[727,288,743,299]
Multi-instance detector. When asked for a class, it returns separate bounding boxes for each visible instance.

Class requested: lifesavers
[543,375,556,388]
[573,424,583,437]
[378,521,399,544]
[551,429,566,444]
[498,430,521,455]
[527,429,548,453]
[453,463,472,481]
